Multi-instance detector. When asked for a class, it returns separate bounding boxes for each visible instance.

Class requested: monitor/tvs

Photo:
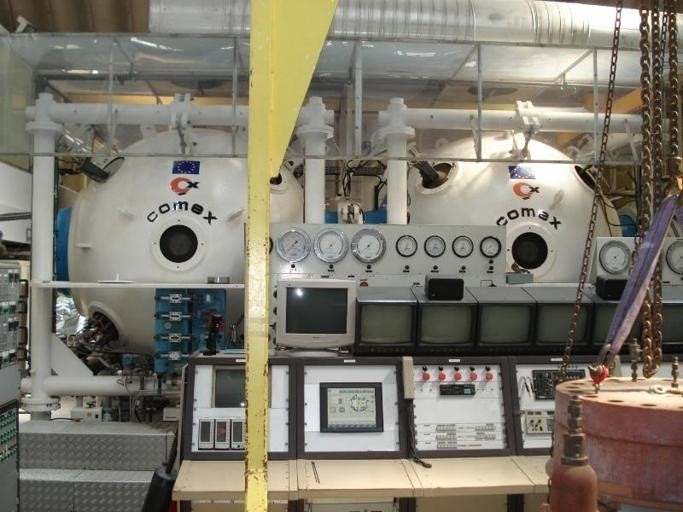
[274,277,357,358]
[468,288,537,356]
[357,285,417,357]
[578,286,645,356]
[411,288,478,354]
[647,300,682,352]
[209,364,253,405]
[524,287,594,355]
